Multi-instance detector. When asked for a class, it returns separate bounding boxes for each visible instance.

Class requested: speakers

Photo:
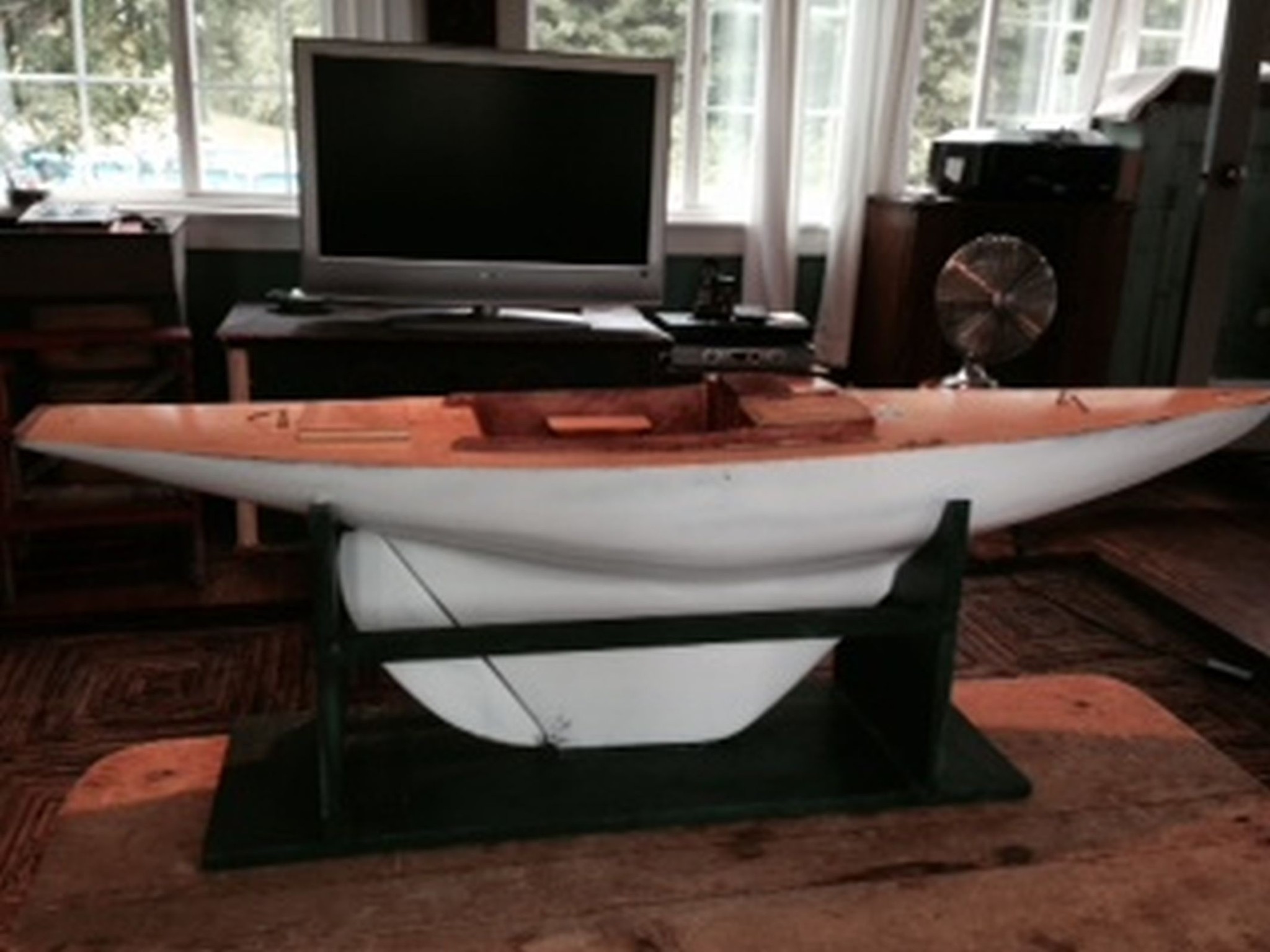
[817,185,1141,385]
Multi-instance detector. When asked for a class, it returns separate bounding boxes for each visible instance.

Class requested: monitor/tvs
[293,38,674,330]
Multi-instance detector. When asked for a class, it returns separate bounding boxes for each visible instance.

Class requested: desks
[219,297,837,562]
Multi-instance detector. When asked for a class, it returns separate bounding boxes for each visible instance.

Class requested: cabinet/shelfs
[0,208,214,602]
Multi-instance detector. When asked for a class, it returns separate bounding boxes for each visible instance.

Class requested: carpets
[11,671,1269,952]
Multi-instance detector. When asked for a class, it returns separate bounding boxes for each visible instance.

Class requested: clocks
[852,192,1137,391]
